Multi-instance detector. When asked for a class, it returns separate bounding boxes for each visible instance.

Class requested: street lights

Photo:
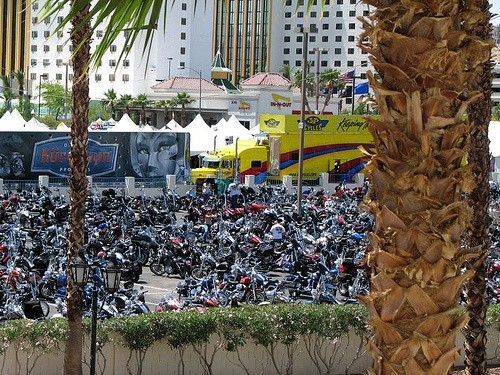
[65,249,125,374]
[177,66,202,116]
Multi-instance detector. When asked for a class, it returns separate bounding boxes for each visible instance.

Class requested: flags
[337,70,354,85]
[354,82,369,94]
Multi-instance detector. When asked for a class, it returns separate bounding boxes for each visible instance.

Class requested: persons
[202,179,212,199]
[130,132,183,183]
[227,179,238,208]
[270,218,286,245]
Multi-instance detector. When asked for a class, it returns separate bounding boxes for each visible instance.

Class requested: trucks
[191,113,469,192]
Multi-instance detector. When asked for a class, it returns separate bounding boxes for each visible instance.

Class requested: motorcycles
[484,180,500,305]
[0,184,374,320]
